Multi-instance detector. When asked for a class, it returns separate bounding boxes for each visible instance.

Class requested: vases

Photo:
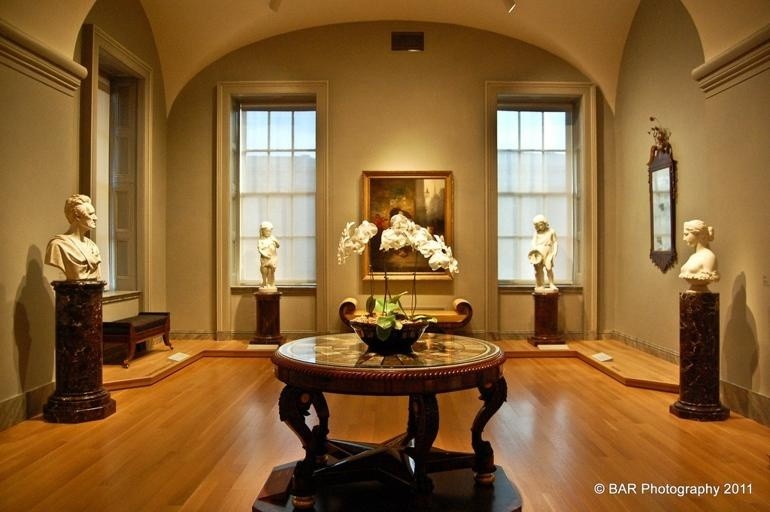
[350,315,434,360]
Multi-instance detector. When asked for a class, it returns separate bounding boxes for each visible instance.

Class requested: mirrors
[646,116,678,274]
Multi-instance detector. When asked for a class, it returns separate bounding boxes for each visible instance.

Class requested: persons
[43,193,105,282]
[256,221,280,288]
[680,218,719,277]
[527,213,561,292]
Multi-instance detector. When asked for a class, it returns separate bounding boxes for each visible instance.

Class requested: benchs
[103,312,174,368]
[339,297,473,333]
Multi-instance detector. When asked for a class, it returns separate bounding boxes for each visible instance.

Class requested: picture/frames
[386,28,427,54]
[362,170,455,281]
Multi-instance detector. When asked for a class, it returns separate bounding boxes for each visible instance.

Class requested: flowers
[337,212,460,342]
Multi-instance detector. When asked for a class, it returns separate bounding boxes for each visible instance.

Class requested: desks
[254,332,524,512]
